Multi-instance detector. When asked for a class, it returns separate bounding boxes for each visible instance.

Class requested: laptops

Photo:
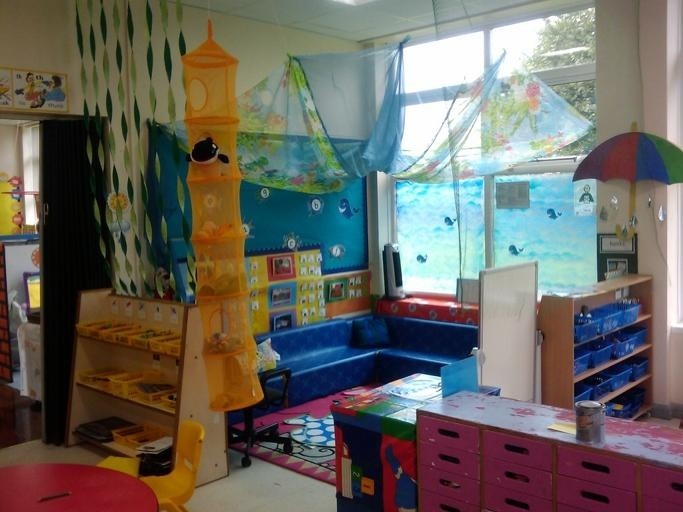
[23,272,40,314]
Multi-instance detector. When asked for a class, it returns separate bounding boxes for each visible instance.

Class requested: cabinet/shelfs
[538,273,653,419]
[64,288,229,489]
[416,392,683,511]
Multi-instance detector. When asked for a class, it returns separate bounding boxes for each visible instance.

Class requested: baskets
[112,423,165,450]
[574,297,649,419]
[77,315,181,356]
[79,366,177,409]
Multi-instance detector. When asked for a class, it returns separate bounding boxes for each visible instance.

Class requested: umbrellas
[573,122,682,241]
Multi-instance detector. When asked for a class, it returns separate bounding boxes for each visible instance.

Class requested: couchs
[230,317,478,427]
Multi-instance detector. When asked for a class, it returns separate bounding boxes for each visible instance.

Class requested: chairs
[139,420,204,512]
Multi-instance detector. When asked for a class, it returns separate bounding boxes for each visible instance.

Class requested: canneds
[575,400,602,443]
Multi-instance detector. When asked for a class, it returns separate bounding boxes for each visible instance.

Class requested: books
[134,435,173,455]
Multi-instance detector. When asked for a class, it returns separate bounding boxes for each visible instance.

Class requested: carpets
[228,383,382,486]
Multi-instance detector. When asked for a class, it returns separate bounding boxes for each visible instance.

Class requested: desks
[0,464,159,512]
[330,372,500,511]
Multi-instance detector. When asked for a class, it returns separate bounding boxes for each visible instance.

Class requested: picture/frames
[1,64,71,113]
